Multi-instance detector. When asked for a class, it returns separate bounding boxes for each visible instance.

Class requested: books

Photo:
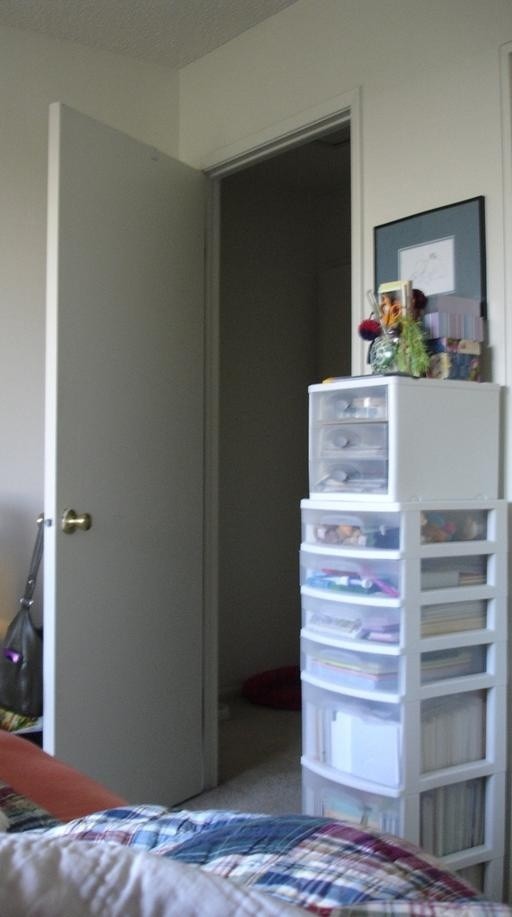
[421,606,489,854]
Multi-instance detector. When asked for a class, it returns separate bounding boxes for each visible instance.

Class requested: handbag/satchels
[0,523,43,717]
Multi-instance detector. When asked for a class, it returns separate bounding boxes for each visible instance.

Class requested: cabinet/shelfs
[299,380,511,903]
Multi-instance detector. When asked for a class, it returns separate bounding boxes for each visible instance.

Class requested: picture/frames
[372,195,486,315]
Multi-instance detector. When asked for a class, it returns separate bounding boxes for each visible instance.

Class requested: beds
[0,786,512,917]
